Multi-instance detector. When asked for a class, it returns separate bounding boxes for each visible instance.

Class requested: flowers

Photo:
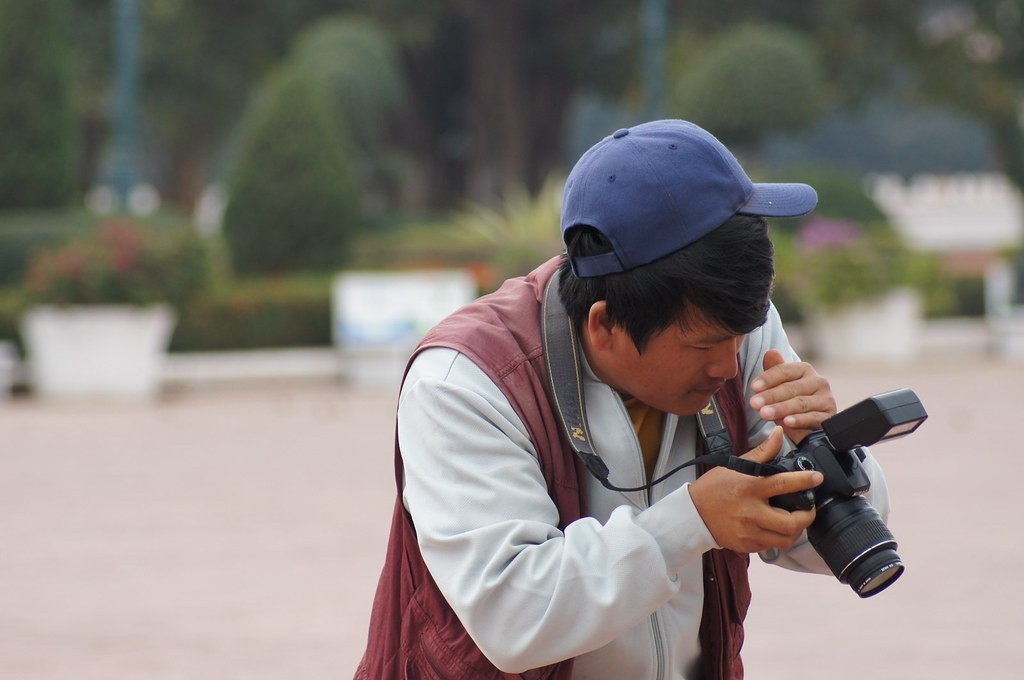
[782,211,932,313]
[17,221,160,305]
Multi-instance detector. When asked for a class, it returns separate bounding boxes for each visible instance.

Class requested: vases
[800,284,924,359]
[16,306,179,401]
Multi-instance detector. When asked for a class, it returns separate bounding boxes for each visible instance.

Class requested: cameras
[760,388,928,600]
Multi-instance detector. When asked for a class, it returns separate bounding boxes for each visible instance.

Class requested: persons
[350,118,888,680]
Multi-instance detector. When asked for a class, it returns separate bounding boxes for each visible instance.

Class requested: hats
[561,119,819,280]
